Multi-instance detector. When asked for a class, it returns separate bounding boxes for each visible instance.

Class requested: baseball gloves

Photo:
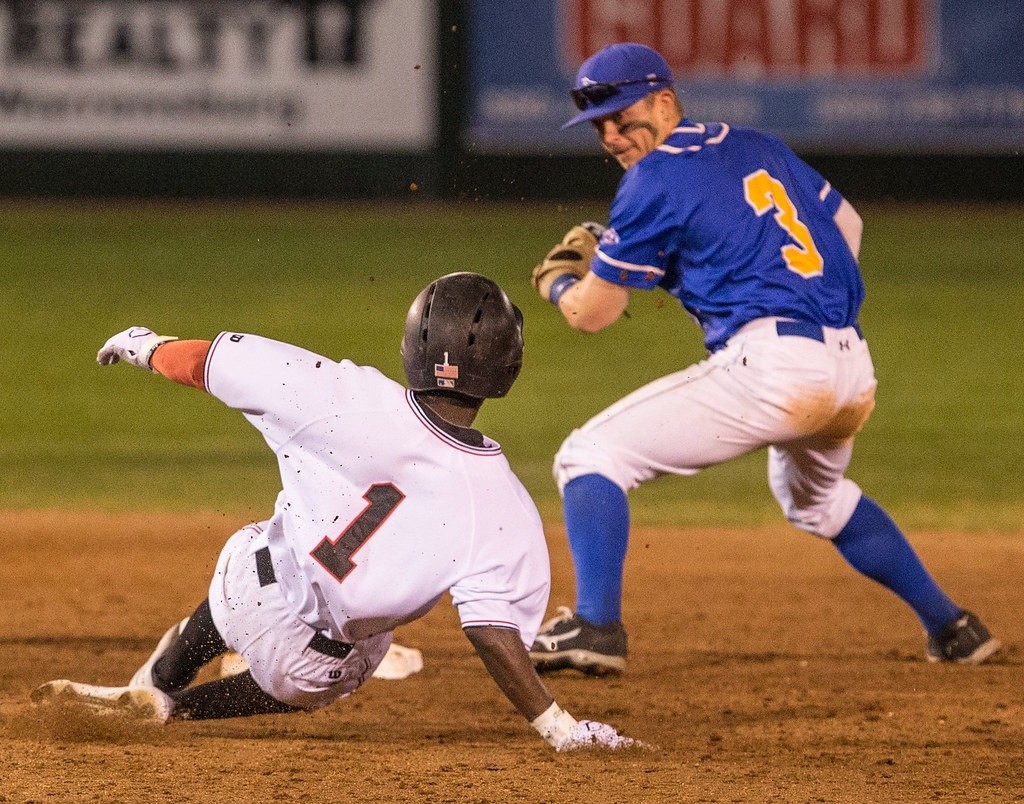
[530,221,608,303]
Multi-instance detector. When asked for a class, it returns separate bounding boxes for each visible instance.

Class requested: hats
[561,43,672,132]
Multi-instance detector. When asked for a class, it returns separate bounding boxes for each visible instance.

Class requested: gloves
[96,326,178,374]
[530,701,659,754]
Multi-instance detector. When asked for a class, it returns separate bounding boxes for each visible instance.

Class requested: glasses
[569,77,669,112]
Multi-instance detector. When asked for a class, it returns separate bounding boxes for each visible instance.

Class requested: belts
[255,547,355,659]
[710,320,864,354]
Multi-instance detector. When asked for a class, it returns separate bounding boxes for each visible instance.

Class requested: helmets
[401,271,522,398]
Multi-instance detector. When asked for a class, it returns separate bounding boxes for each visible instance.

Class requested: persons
[28,271,641,755]
[521,42,1003,676]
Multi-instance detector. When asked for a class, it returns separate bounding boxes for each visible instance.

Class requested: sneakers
[29,677,176,727]
[925,609,1003,668]
[526,605,629,677]
[131,615,193,688]
